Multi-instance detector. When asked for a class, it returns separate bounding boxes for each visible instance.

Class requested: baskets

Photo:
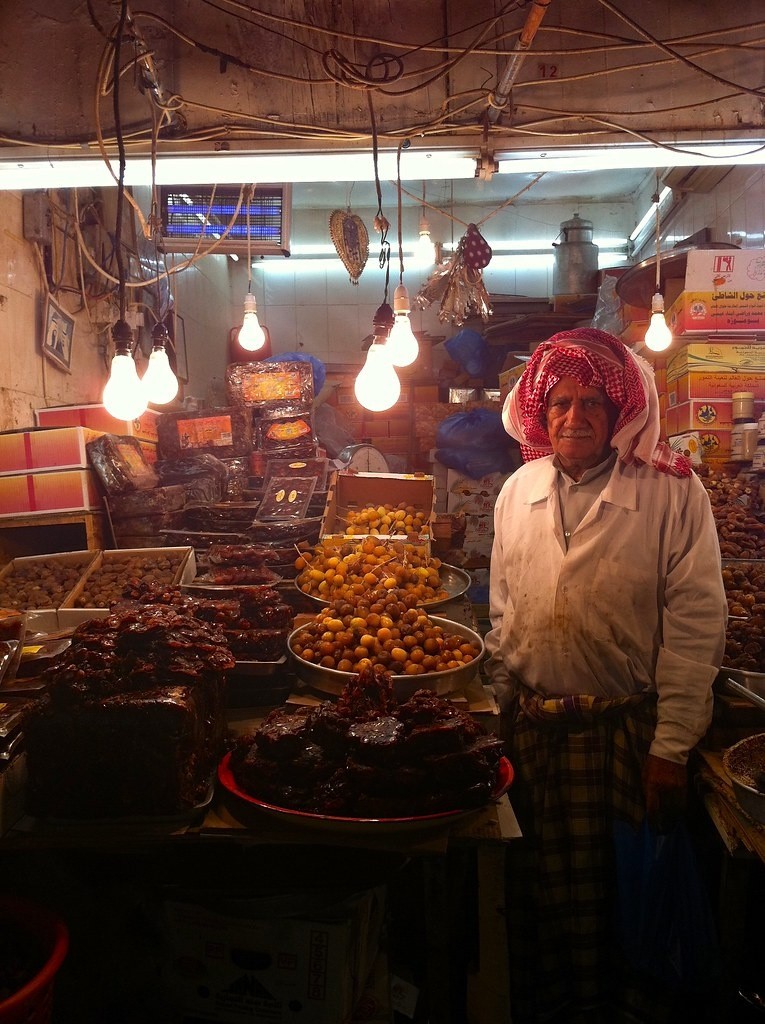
[0,895,70,1024]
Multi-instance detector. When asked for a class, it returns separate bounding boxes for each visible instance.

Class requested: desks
[7,704,525,1024]
[690,774,765,1024]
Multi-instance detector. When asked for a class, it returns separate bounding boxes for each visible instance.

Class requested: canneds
[729,391,765,507]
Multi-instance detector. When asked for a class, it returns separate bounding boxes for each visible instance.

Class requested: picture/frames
[40,291,77,375]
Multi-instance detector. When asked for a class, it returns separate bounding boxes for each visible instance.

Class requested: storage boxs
[0,249,765,1024]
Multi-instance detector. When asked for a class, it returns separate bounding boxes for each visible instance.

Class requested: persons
[481,326,729,1023]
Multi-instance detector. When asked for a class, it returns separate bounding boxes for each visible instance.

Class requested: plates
[615,244,741,309]
[296,561,473,611]
[219,748,514,830]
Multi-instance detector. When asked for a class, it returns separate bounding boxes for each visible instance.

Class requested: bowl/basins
[724,734,765,819]
[713,667,765,694]
[288,615,486,699]
